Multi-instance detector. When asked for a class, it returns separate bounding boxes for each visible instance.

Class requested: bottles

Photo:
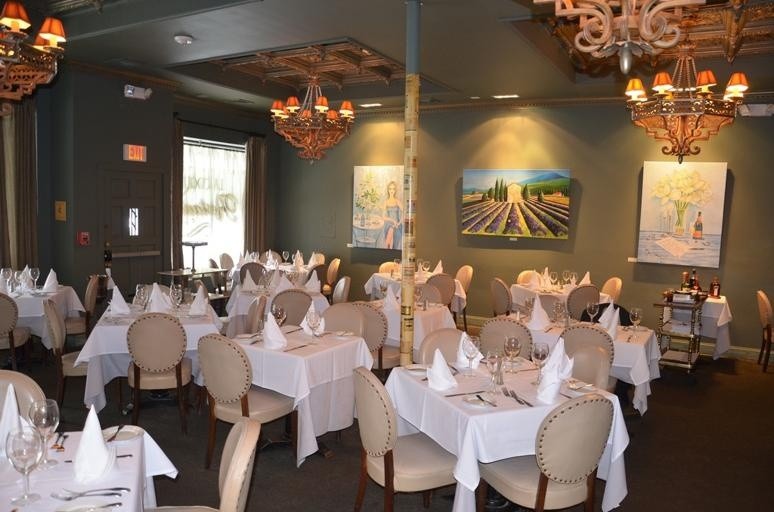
[680,268,720,299]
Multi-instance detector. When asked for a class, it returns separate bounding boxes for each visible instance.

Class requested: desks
[668,293,729,356]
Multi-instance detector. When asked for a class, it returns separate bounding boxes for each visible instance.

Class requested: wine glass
[391,259,433,271]
[245,249,304,269]
[548,269,579,289]
[505,336,521,373]
[377,279,389,301]
[5,426,44,507]
[136,282,184,317]
[484,351,501,394]
[29,399,59,469]
[267,302,324,346]
[522,295,647,341]
[415,287,423,304]
[530,343,549,384]
[462,336,480,371]
[1,267,45,296]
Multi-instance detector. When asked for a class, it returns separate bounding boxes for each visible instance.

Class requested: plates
[102,425,144,443]
[328,329,354,340]
[235,334,255,341]
[463,394,496,407]
[567,381,600,394]
[57,500,111,512]
[404,365,434,375]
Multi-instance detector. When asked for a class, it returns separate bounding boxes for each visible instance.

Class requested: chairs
[755,288,774,373]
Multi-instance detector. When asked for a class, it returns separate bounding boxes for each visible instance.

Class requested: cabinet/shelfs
[652,296,707,374]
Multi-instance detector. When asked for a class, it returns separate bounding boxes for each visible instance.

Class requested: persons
[381,179,405,250]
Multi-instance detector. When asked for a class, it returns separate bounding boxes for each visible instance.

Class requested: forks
[57,435,69,452]
[501,387,524,406]
[51,431,63,450]
[50,487,131,501]
[510,390,533,407]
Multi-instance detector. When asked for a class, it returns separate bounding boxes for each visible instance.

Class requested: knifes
[282,343,312,353]
[250,338,264,346]
[286,327,304,334]
[445,390,485,398]
[62,488,122,498]
[65,454,132,462]
[107,425,124,442]
[477,395,496,408]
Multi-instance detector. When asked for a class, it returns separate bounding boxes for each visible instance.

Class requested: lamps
[623,39,749,165]
[1,2,68,100]
[269,65,357,160]
